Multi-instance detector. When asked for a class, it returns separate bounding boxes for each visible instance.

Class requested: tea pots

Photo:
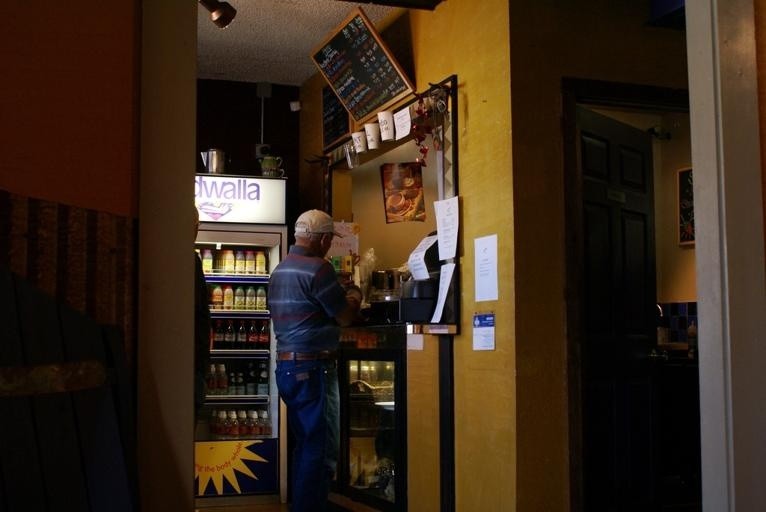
[200,146,231,174]
[261,154,285,177]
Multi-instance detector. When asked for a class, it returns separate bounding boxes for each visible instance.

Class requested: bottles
[211,408,271,436]
[688,320,698,361]
[214,320,269,350]
[209,284,266,310]
[331,255,352,273]
[207,360,269,395]
[195,249,266,274]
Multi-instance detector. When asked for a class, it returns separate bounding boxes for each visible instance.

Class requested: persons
[268,209,364,512]
[192,206,213,415]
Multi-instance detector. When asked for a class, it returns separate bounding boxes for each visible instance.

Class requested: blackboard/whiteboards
[308,8,416,125]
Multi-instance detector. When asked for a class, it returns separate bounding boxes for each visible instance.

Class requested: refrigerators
[191,223,289,503]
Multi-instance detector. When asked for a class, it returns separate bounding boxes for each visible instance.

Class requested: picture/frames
[675,166,696,248]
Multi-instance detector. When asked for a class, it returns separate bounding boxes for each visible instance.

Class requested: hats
[294,207,345,238]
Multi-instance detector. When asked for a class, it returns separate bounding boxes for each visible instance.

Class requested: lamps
[200,0,238,29]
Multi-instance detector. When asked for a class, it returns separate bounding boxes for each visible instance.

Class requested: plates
[399,199,412,216]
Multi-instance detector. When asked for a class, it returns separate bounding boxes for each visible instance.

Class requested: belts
[277,349,335,361]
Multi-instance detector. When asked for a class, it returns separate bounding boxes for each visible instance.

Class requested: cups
[386,192,406,211]
[344,111,394,169]
